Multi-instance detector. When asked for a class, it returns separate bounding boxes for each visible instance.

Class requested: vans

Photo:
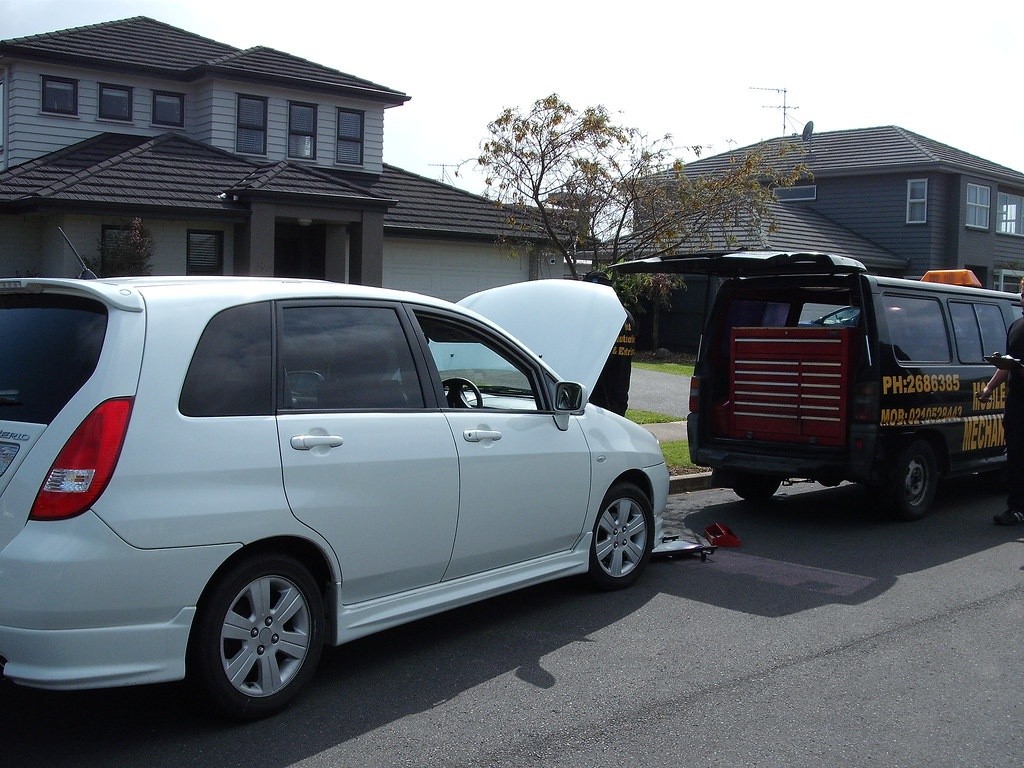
[600,249,1024,521]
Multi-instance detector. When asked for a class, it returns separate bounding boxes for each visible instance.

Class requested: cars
[0,275,673,728]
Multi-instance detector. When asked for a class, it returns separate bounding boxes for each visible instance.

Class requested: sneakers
[994,508,1024,525]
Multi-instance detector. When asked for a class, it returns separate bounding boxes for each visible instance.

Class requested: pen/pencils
[978,398,992,401]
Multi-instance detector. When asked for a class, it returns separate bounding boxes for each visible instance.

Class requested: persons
[974,277,1024,526]
[584,270,637,417]
